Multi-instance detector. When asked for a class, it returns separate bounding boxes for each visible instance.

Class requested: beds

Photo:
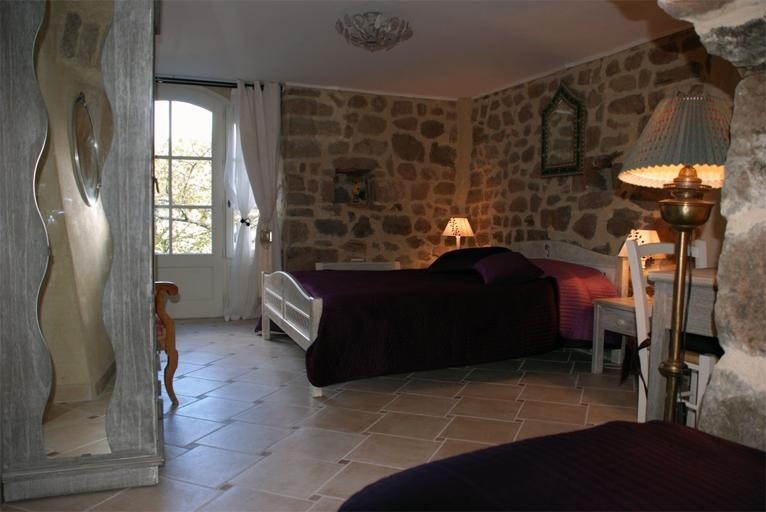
[260,239,629,398]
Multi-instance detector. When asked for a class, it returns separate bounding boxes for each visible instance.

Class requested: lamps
[619,228,667,300]
[614,93,735,424]
[442,217,475,252]
[334,10,414,53]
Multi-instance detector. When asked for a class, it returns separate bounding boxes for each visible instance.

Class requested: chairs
[625,237,723,426]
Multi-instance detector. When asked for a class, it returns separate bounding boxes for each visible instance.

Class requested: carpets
[336,419,765,512]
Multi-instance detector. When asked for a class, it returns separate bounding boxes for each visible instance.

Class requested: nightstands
[591,297,654,376]
